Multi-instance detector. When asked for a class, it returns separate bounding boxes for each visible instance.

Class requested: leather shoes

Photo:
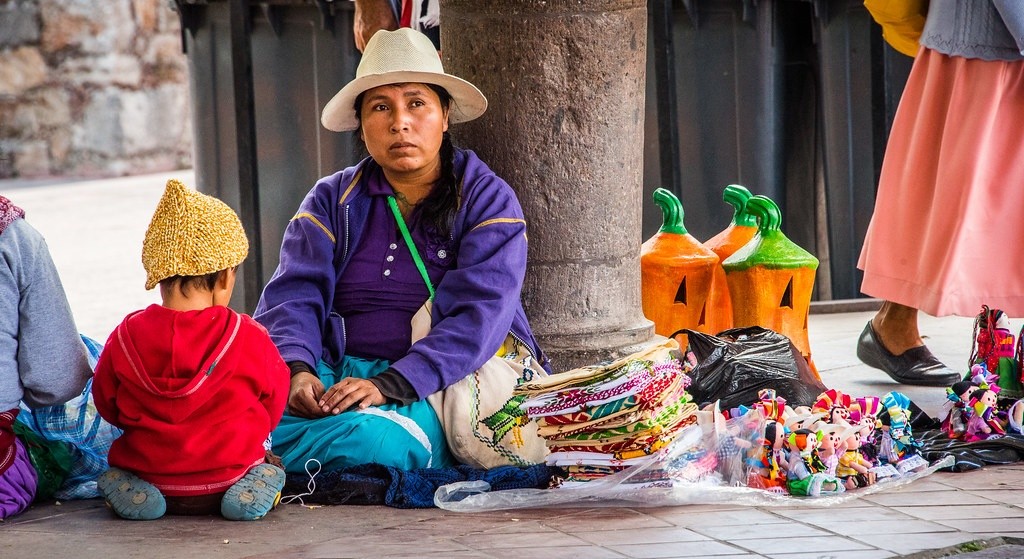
[857,320,961,387]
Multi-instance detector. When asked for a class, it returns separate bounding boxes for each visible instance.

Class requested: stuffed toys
[724,387,924,497]
[940,306,1024,442]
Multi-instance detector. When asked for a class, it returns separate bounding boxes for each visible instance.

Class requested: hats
[319,27,488,132]
[142,178,249,291]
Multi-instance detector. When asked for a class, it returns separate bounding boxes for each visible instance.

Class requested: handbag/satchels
[668,325,827,423]
[410,298,551,471]
[863,0,928,58]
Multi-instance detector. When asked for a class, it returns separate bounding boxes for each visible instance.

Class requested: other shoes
[220,463,287,521]
[98,470,167,520]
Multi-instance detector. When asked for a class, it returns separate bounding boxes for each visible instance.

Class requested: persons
[855,1,1023,390]
[352,0,441,64]
[90,179,291,522]
[0,196,94,526]
[248,25,551,487]
